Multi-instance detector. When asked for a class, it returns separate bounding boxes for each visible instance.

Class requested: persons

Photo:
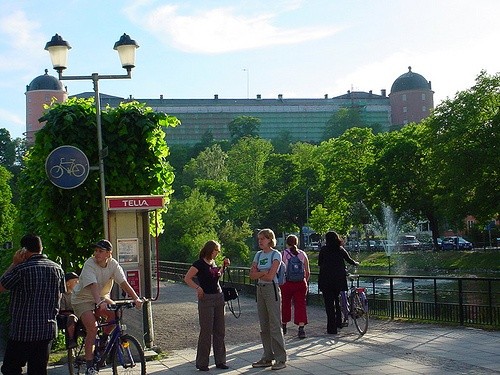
[250,228,286,369]
[185,240,229,371]
[71,240,143,375]
[59,272,79,349]
[318,232,359,334]
[0,233,67,375]
[281,235,310,337]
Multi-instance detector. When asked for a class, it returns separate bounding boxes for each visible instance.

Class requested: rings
[15,251,18,254]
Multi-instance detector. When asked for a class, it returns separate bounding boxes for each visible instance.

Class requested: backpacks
[284,249,305,282]
[256,250,287,303]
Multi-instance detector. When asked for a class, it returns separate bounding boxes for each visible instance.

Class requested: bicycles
[67,297,149,375]
[332,265,370,335]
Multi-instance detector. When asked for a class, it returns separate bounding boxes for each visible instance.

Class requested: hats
[65,272,79,282]
[93,239,113,251]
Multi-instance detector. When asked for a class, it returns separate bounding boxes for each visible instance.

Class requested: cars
[344,234,421,252]
[440,236,473,251]
[308,242,318,251]
[418,238,444,250]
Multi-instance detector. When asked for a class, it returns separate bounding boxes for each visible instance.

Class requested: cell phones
[21,247,27,253]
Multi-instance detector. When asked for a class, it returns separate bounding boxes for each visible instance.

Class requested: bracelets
[196,286,199,289]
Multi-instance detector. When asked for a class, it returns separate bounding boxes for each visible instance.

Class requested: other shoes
[67,339,79,349]
[282,324,286,334]
[200,367,210,371]
[298,328,306,338]
[86,367,99,375]
[216,364,229,369]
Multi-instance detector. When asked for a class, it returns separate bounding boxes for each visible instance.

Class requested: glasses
[214,249,221,254]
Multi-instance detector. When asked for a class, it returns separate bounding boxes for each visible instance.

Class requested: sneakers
[252,359,272,367]
[271,361,286,370]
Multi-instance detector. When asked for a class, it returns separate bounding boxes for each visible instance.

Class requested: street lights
[44,32,140,243]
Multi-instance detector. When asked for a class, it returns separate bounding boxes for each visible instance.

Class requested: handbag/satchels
[222,261,241,319]
